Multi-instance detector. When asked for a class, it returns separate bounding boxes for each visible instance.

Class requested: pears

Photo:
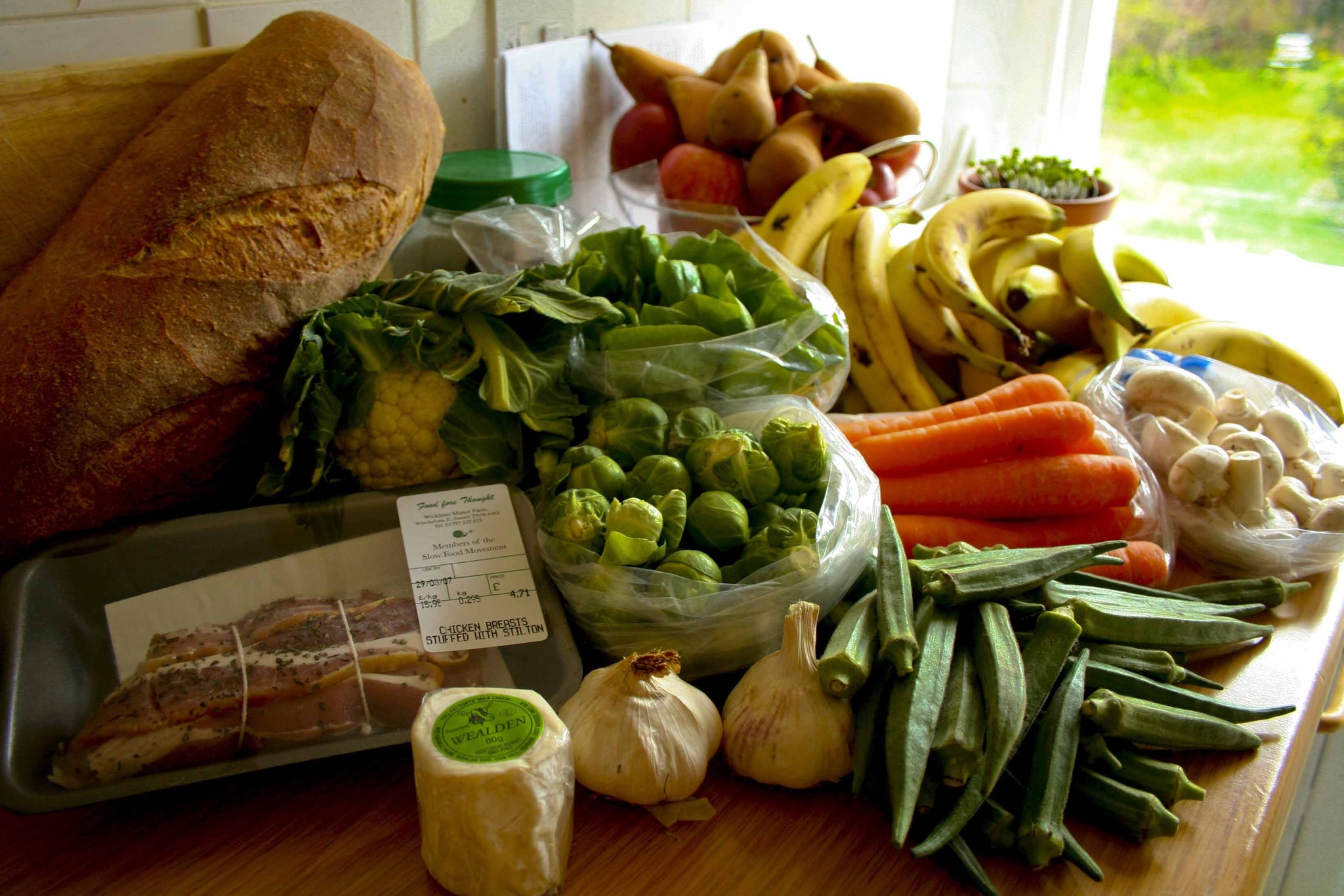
[589,28,921,216]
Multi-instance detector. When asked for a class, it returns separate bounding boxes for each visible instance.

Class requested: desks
[1,560,1343,895]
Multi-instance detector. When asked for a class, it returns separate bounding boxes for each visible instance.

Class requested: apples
[656,141,753,215]
[854,189,883,206]
[864,158,899,202]
[609,101,682,172]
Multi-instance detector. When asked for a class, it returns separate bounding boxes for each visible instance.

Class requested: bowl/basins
[609,160,925,238]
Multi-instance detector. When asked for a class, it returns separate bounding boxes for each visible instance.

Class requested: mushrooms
[1115,366,1344,571]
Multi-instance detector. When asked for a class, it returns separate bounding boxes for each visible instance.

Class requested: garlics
[554,647,722,804]
[722,602,856,790]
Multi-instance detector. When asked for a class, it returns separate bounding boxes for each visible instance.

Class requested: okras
[818,505,1315,896]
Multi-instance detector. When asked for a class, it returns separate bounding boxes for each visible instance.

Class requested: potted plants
[960,147,1118,227]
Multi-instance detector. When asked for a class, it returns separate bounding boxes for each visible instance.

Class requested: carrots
[822,372,1168,588]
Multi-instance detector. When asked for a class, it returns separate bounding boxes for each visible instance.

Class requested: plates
[0,478,583,814]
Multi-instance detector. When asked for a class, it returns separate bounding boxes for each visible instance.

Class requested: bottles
[392,149,572,278]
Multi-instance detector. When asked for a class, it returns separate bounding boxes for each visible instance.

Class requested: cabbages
[545,398,832,620]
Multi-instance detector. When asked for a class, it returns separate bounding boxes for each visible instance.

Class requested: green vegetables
[554,222,847,416]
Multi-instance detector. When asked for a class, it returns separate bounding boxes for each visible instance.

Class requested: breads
[1,9,446,557]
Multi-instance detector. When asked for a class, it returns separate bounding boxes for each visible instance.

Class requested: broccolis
[263,260,620,498]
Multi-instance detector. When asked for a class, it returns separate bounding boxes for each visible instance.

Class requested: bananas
[732,154,1343,427]
